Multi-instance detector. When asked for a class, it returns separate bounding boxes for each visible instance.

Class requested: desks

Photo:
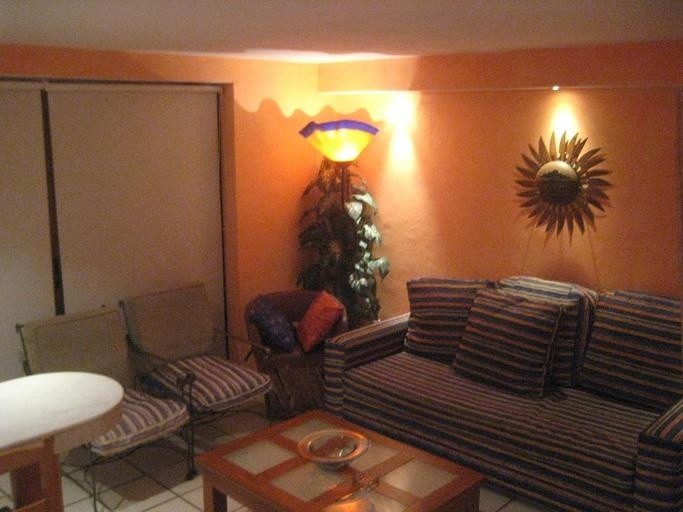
[188,410,492,511]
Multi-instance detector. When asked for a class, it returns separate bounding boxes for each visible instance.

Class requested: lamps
[299,112,380,205]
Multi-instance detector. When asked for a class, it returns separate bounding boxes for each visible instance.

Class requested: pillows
[402,274,601,398]
[249,298,294,347]
[295,287,346,352]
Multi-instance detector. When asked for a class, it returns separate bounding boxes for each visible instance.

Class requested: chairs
[244,287,352,423]
[11,303,196,512]
[116,278,276,482]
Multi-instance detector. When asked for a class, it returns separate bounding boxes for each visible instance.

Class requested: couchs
[318,267,680,511]
